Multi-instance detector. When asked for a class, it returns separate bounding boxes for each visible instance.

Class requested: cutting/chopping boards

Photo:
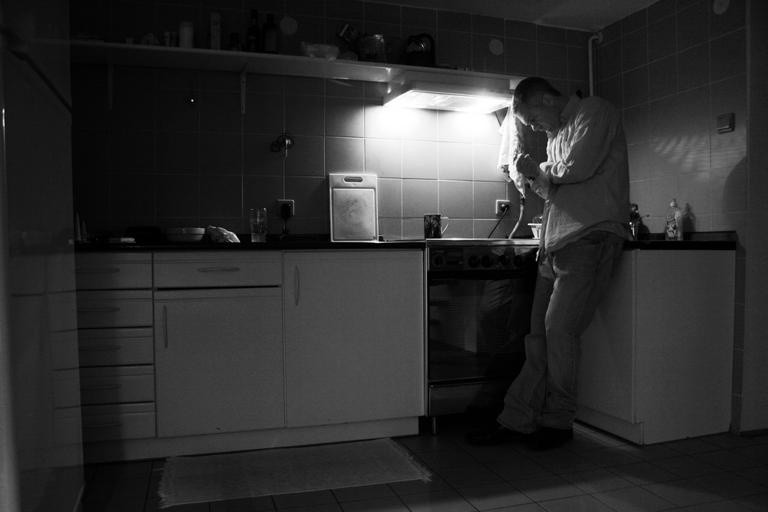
[329,173,379,243]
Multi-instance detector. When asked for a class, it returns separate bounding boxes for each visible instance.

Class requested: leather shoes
[466,425,573,449]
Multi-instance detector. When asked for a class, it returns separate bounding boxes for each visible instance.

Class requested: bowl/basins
[303,44,339,61]
[182,227,205,241]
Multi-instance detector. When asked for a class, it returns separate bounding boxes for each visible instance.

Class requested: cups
[249,208,266,243]
[424,214,449,238]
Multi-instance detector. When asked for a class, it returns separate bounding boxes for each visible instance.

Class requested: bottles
[665,198,684,242]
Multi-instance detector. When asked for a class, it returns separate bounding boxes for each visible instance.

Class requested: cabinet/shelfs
[78,247,427,464]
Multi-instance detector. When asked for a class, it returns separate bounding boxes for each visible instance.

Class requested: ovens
[425,243,540,418]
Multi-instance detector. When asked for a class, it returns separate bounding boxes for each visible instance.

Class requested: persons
[464,76,634,450]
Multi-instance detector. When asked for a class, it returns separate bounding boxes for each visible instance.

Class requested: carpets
[153,435,429,506]
